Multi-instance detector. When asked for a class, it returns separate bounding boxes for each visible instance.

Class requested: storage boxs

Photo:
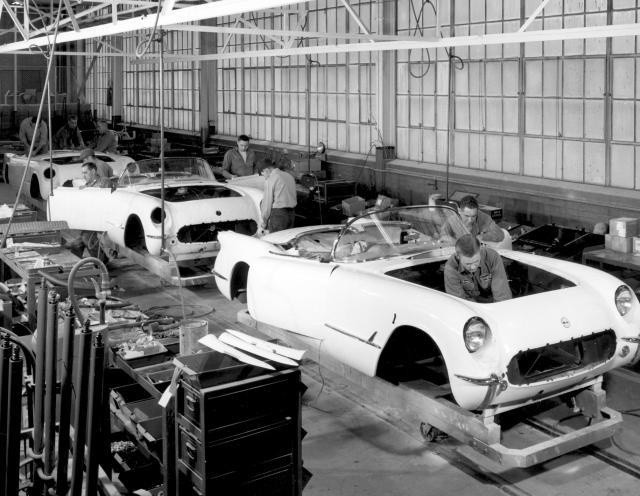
[340,194,366,217]
[602,217,640,257]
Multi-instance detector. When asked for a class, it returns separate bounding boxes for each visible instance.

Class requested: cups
[179,320,209,358]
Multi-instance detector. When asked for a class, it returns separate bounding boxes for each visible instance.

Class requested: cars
[214,205,640,415]
[3,147,263,262]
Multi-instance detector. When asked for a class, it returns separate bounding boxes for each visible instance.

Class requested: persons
[18,116,48,157]
[443,233,513,304]
[79,149,113,178]
[94,120,118,155]
[69,162,118,271]
[222,135,256,197]
[441,195,504,245]
[55,115,85,150]
[255,157,298,233]
